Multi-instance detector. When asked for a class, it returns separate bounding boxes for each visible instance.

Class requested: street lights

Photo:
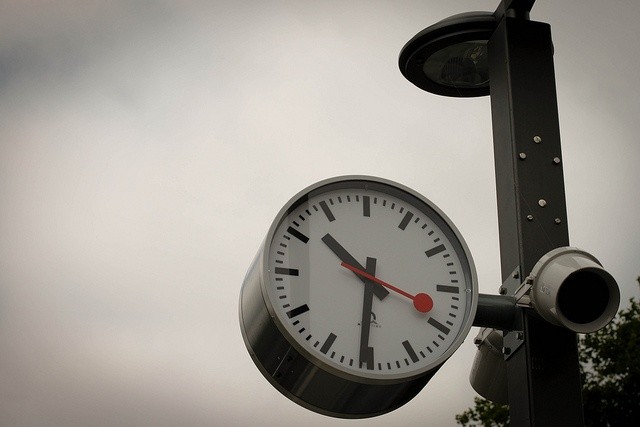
[398,1,578,427]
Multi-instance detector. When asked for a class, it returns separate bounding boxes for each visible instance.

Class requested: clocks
[239,173,480,419]
